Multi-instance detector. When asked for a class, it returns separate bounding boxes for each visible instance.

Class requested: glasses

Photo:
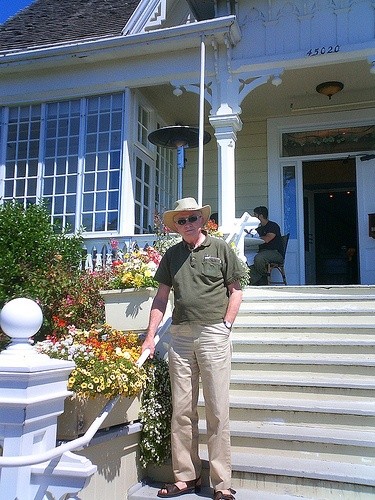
[175,215,198,225]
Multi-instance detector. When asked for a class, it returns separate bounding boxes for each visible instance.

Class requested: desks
[244,236,265,285]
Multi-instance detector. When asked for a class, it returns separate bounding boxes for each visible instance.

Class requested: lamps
[317,81,344,100]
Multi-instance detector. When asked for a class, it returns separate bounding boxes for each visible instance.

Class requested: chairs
[267,233,290,285]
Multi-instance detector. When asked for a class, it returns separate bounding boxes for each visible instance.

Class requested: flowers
[33,217,250,467]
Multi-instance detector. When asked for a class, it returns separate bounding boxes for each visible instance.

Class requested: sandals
[157,478,201,498]
[213,487,236,500]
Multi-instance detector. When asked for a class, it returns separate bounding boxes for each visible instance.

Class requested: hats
[163,197,211,230]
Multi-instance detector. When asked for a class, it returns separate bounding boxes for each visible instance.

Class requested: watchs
[224,320,232,330]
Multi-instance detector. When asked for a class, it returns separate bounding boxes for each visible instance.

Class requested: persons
[246,206,284,286]
[209,212,218,229]
[140,198,245,500]
